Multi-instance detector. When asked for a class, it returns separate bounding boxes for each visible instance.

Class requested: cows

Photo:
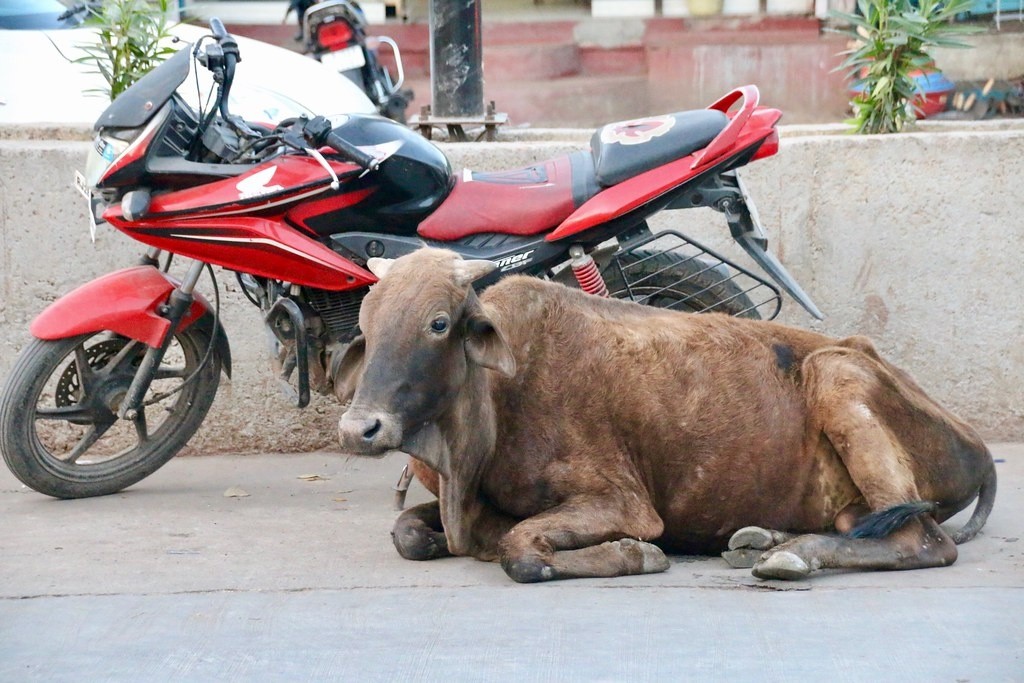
[333,245,999,586]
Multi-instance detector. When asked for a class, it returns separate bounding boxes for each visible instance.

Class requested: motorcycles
[280,0,414,124]
[0,17,826,498]
